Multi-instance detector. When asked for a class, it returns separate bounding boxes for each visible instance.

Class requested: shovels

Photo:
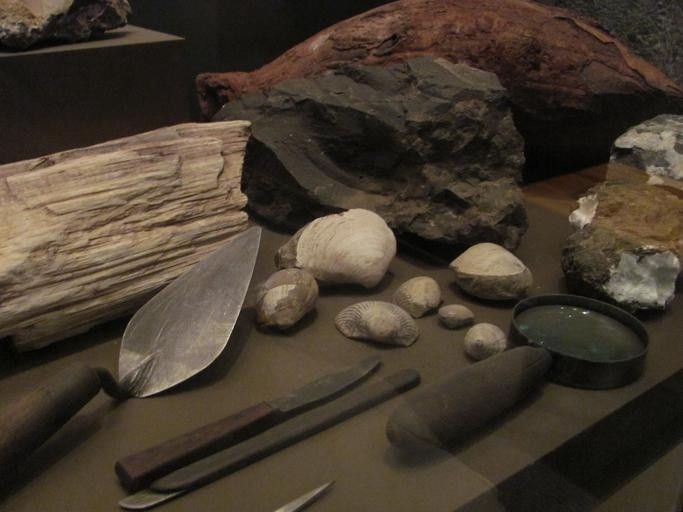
[0,226,262,478]
